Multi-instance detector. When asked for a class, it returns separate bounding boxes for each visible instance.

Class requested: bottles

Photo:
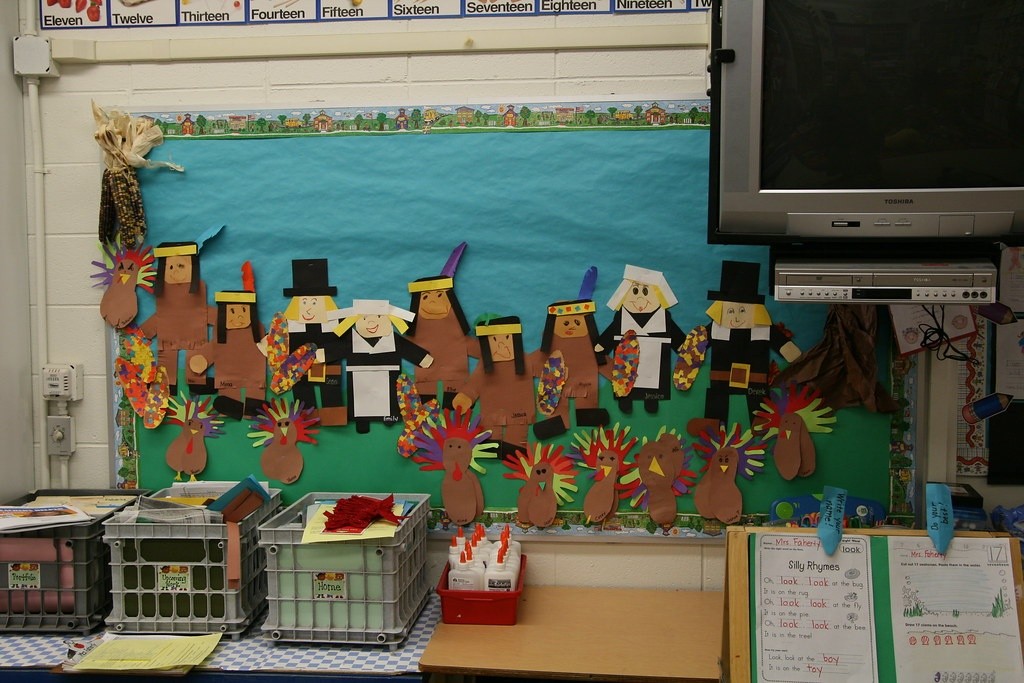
[484,524,522,591]
[448,523,492,590]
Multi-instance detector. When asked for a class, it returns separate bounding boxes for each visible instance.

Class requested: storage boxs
[0,488,153,638]
[101,487,282,641]
[257,492,431,653]
[437,554,528,626]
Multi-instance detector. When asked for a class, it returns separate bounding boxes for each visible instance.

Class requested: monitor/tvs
[717,0,1024,242]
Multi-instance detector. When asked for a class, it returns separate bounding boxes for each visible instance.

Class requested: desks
[0,589,725,683]
